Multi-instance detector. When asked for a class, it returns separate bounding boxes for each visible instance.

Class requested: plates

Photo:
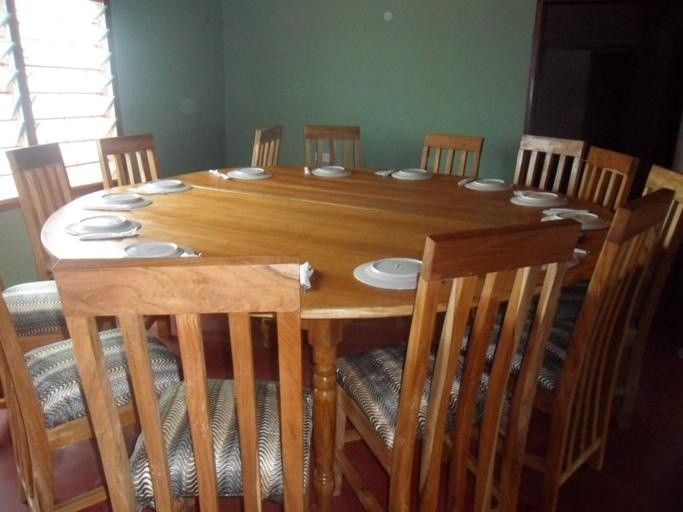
[508,191,569,208]
[539,210,608,232]
[63,214,195,258]
[310,163,353,178]
[226,165,274,181]
[141,178,192,194]
[391,167,432,182]
[351,257,422,290]
[461,177,514,193]
[91,191,153,209]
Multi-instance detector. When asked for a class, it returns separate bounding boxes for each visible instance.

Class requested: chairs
[95,132,162,190]
[49,255,311,510]
[418,132,483,178]
[530,163,683,429]
[445,188,674,510]
[331,217,581,510]
[7,143,169,340]
[251,125,283,167]
[572,145,639,290]
[512,135,588,197]
[302,124,360,169]
[0,294,184,511]
